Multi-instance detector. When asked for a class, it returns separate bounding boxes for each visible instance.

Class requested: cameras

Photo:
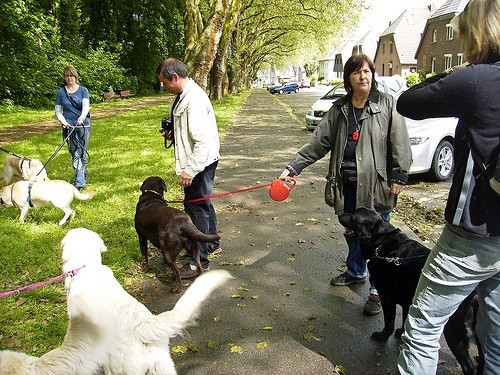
[162,119,171,130]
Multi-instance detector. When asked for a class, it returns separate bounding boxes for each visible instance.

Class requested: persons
[279,54,413,315]
[55,65,92,188]
[156,57,223,280]
[394,0,500,375]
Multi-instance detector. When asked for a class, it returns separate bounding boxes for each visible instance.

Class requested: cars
[269,82,299,94]
[391,116,462,182]
[304,75,410,134]
[301,81,310,88]
[266,82,281,91]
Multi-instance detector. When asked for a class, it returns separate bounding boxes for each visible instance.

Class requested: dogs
[337,207,484,375]
[0,153,94,226]
[135,176,221,293]
[0,228,235,375]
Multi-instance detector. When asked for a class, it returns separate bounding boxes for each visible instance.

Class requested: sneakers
[364,294,381,313]
[209,248,223,255]
[330,272,366,286]
[178,262,210,278]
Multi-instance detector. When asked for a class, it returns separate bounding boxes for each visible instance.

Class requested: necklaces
[352,102,363,131]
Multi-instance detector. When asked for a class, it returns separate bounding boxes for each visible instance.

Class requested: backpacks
[467,132,500,238]
